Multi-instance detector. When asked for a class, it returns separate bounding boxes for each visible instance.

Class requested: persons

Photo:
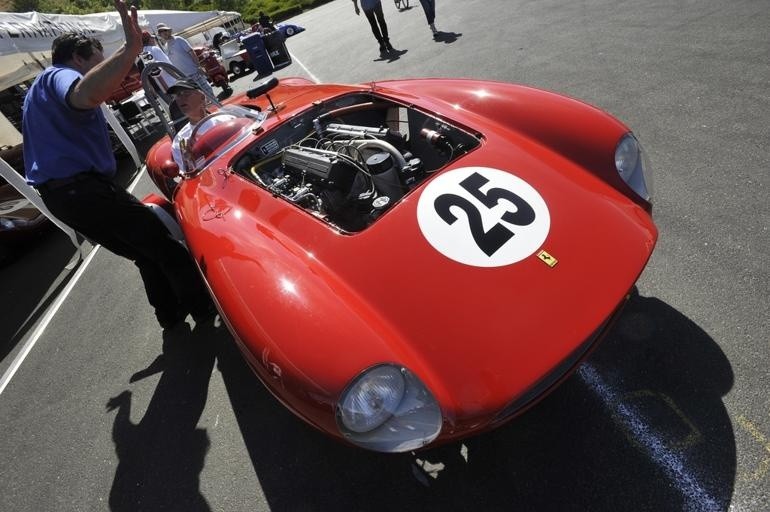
[259,11,271,28]
[166,79,236,179]
[139,30,177,97]
[418,0,438,38]
[352,0,392,57]
[156,23,215,105]
[212,32,224,56]
[20,1,231,362]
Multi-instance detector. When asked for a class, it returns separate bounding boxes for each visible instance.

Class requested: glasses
[175,91,200,98]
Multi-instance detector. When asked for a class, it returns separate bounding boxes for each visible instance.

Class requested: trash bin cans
[262,30,291,67]
[242,32,273,75]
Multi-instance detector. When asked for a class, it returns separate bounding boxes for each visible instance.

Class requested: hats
[167,79,202,94]
[142,23,173,42]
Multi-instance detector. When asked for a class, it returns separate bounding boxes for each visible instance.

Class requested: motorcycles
[195,48,230,95]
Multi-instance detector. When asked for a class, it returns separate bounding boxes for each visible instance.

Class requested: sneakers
[162,322,204,371]
[378,38,391,51]
[429,23,437,35]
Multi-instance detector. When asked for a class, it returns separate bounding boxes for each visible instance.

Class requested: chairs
[106,100,160,156]
[169,98,210,163]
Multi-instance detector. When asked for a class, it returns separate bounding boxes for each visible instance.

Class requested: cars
[203,22,305,77]
[1,139,47,233]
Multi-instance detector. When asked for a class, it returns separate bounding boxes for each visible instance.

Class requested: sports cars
[139,58,661,451]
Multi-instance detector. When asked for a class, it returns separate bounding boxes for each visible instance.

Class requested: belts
[38,172,96,194]
